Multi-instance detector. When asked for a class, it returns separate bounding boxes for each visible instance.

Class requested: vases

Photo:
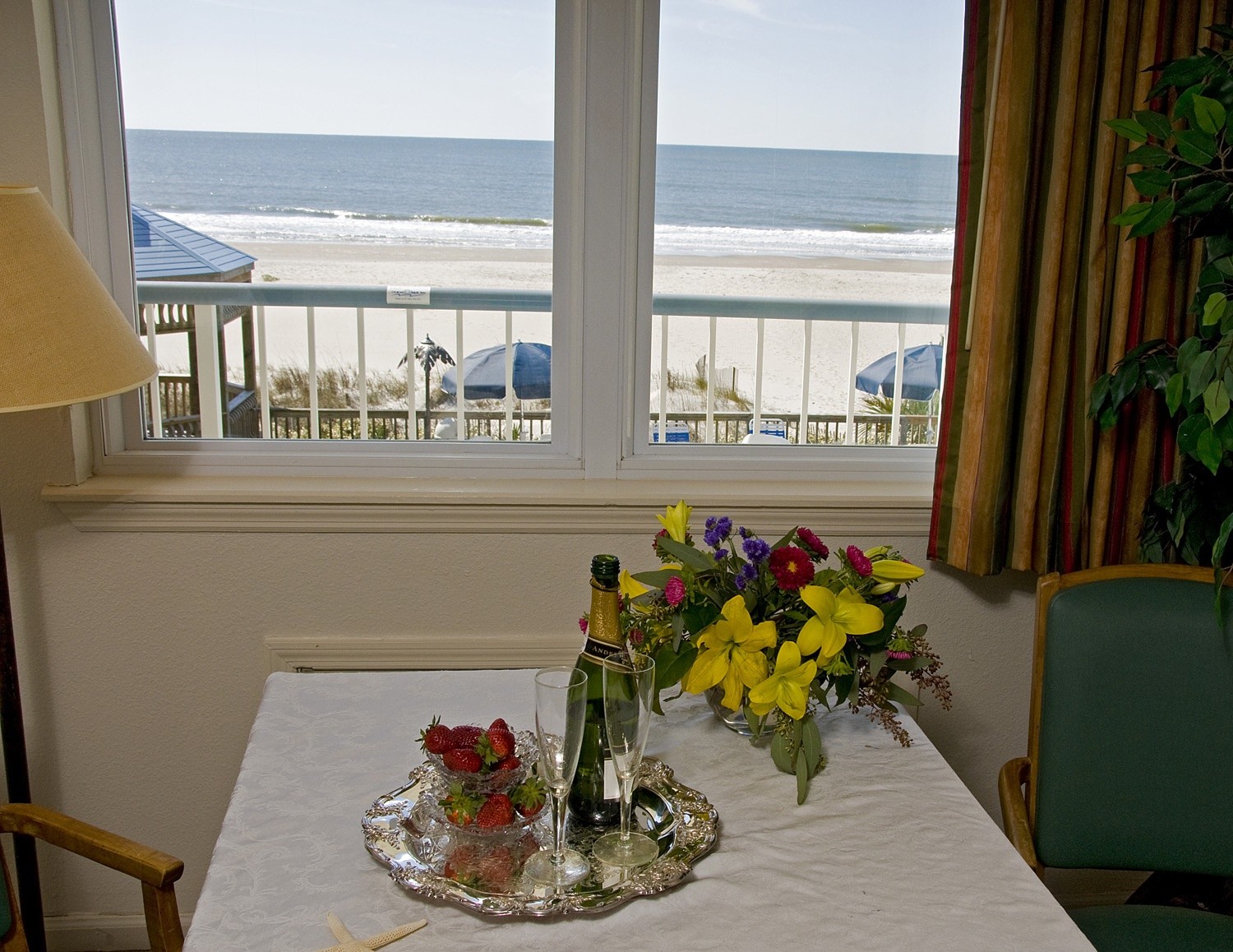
[707,683,794,738]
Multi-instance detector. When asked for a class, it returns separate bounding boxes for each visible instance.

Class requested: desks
[178,669,1100,952]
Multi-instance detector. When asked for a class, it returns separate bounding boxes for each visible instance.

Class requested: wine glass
[524,665,591,887]
[591,652,659,868]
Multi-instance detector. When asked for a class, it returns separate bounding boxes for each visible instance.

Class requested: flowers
[576,499,953,805]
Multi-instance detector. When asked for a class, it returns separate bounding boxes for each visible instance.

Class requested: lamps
[0,182,163,952]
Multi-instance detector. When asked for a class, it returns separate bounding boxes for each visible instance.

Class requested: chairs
[996,564,1233,952]
[652,422,690,444]
[0,803,187,952]
[748,418,786,437]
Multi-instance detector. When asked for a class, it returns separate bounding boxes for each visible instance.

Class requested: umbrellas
[438,338,551,440]
[855,342,943,444]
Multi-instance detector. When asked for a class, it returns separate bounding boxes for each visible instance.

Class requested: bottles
[562,554,639,826]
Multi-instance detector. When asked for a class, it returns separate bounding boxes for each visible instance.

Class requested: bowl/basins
[424,721,539,794]
[418,781,551,836]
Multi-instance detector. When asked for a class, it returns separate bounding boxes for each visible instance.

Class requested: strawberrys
[415,715,522,787]
[438,762,546,829]
[445,833,541,899]
